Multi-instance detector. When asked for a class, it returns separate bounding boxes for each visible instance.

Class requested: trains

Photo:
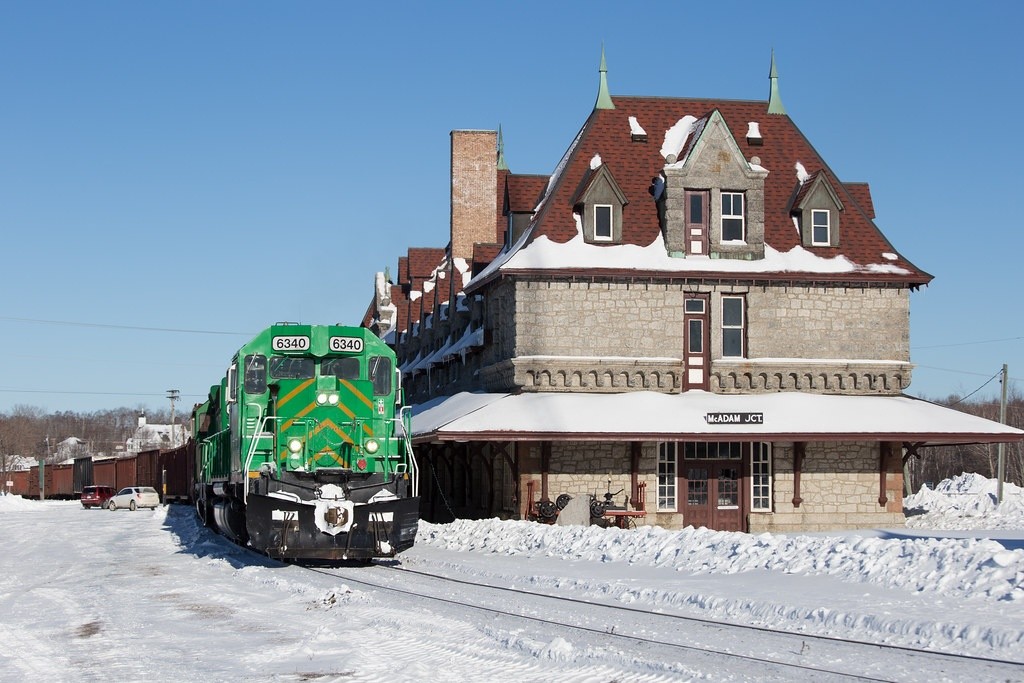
[0,322,421,568]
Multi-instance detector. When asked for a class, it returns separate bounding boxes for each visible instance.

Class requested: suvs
[108,486,159,511]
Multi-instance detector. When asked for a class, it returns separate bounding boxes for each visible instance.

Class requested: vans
[81,485,118,508]
